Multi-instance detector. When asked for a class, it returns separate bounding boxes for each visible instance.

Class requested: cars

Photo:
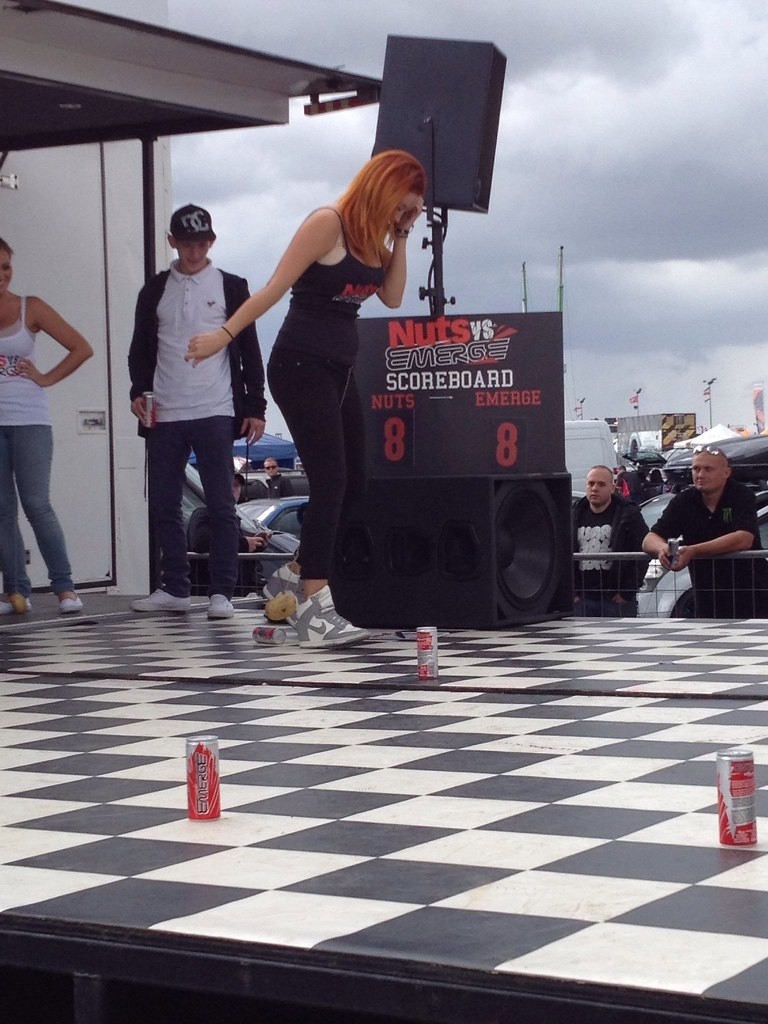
[571,433,768,619]
[150,461,311,599]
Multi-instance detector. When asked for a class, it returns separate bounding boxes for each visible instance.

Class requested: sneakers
[0,596,33,614]
[58,596,83,616]
[295,593,371,649]
[131,589,194,612]
[207,595,235,620]
[263,565,299,628]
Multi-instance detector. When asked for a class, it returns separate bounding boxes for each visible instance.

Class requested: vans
[564,419,616,493]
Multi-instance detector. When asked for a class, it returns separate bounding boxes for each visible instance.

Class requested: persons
[642,445,768,618]
[264,457,295,498]
[187,472,272,596]
[184,149,428,649]
[127,203,268,619]
[0,238,94,617]
[572,466,650,618]
[616,465,641,505]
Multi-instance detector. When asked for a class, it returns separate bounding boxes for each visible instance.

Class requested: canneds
[416,626,439,680]
[668,538,680,566]
[185,735,221,822]
[716,749,758,848]
[141,392,157,429]
[253,626,287,644]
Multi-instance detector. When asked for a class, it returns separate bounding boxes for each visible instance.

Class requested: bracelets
[395,228,409,238]
[221,326,235,339]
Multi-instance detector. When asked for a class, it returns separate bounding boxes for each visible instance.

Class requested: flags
[572,402,581,416]
[704,386,710,402]
[629,394,638,409]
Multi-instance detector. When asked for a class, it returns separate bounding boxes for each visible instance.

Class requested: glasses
[263,465,278,470]
[693,445,728,460]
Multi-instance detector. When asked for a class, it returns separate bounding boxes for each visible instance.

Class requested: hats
[232,471,246,486]
[169,204,218,245]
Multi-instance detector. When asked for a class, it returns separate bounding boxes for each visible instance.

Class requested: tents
[674,424,741,448]
[188,432,298,469]
